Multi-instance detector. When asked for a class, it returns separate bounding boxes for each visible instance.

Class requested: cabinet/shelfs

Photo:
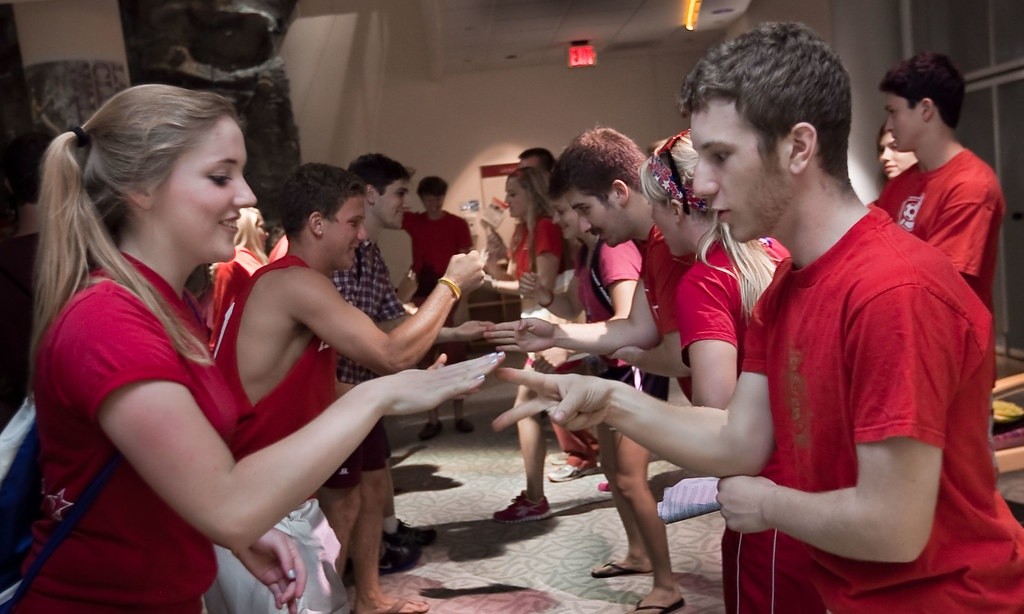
[462,259,521,348]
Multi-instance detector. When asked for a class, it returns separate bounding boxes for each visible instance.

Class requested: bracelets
[439,277,461,299]
[538,289,554,308]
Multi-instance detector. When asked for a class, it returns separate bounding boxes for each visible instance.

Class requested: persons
[866,119,919,205]
[483,124,790,614]
[0,130,54,432]
[868,53,1008,388]
[14,84,508,613]
[491,24,1024,614]
[200,152,472,576]
[215,161,488,614]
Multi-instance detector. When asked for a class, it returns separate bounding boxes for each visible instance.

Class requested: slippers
[349,597,426,614]
[634,597,684,614]
[591,561,654,578]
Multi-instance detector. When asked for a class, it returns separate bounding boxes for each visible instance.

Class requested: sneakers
[552,455,567,465]
[493,490,553,524]
[377,540,422,575]
[547,463,599,483]
[597,480,612,492]
[381,518,437,549]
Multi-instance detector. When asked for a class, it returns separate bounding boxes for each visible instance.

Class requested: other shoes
[455,419,475,433]
[419,420,441,441]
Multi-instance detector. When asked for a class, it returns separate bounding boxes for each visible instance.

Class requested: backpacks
[0,276,175,614]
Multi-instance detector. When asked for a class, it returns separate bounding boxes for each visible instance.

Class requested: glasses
[658,127,691,215]
